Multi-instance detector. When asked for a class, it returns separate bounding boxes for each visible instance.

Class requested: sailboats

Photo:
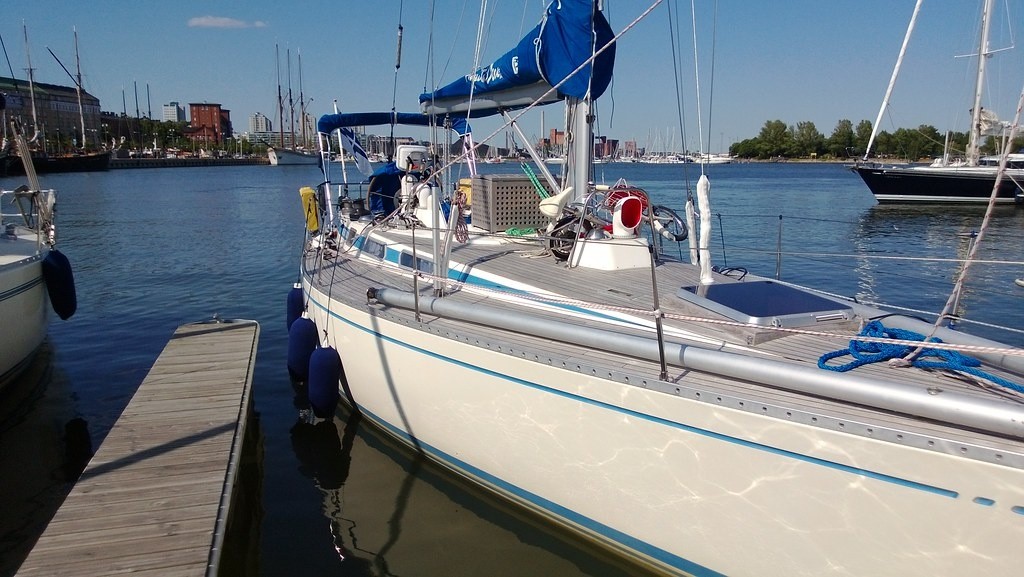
[0,18,114,178]
[259,43,320,166]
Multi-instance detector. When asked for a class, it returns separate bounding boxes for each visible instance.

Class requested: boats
[0,115,78,392]
[280,1,1024,577]
[851,0,1024,209]
